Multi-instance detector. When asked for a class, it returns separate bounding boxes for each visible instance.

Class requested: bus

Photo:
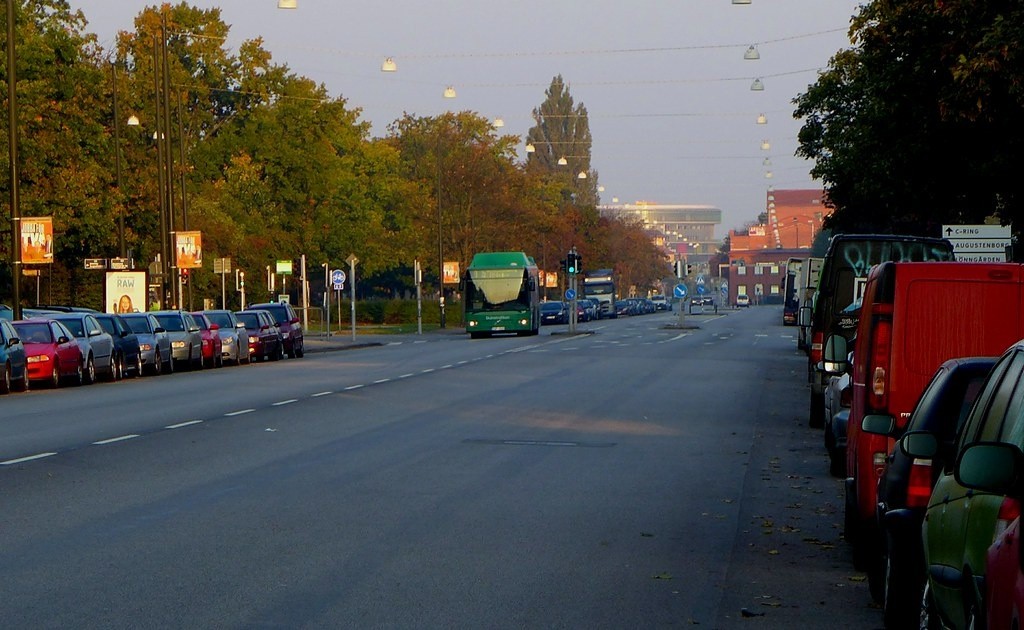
[458,251,541,339]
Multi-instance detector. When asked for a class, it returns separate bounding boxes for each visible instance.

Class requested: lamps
[756,114,768,124]
[761,140,770,150]
[750,79,764,90]
[767,185,774,192]
[765,172,773,179]
[744,45,760,59]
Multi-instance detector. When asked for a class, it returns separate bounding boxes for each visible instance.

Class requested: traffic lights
[577,256,582,271]
[674,261,681,279]
[567,254,575,273]
[181,268,189,282]
[560,260,566,273]
[270,295,274,304]
[684,264,691,277]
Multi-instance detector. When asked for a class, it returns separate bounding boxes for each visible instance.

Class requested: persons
[193,244,201,264]
[41,233,52,258]
[113,295,133,313]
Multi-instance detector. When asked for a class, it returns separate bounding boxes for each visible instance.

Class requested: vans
[736,295,750,308]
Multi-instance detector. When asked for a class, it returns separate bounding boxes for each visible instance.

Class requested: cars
[615,295,673,316]
[540,298,601,325]
[0,304,305,396]
[861,339,1024,630]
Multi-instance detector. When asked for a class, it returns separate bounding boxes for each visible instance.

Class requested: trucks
[781,234,1024,547]
[582,269,617,319]
